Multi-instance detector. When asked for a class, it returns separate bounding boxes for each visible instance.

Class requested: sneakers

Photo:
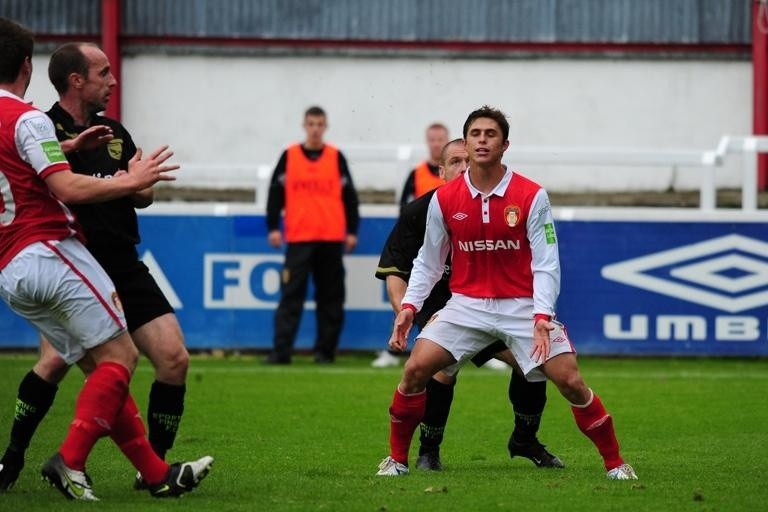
[368,348,401,369]
[375,454,411,477]
[38,451,103,504]
[506,432,565,470]
[607,464,639,481]
[413,452,444,474]
[135,454,216,500]
[0,447,22,495]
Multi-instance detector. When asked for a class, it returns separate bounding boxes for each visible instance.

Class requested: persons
[265,106,360,364]
[0,39,189,498]
[372,123,508,369]
[374,137,565,473]
[375,106,637,481]
[0,20,215,501]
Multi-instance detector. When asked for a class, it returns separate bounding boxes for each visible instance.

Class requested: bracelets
[401,304,418,316]
[534,315,550,324]
